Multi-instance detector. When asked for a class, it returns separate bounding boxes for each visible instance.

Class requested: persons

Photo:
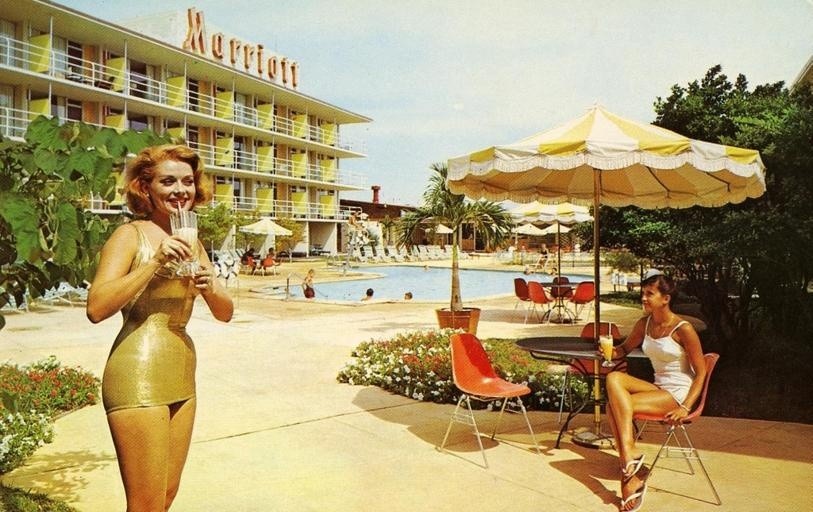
[87,145,234,511]
[348,211,359,227]
[596,274,706,512]
[302,269,315,298]
[361,288,375,301]
[257,248,280,270]
[241,248,256,275]
[404,292,413,301]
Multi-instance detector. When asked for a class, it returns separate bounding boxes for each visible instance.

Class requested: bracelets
[680,403,693,416]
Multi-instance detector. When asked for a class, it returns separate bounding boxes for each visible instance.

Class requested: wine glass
[169,210,203,277]
[599,335,616,368]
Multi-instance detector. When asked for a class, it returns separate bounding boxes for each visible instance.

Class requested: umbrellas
[239,217,293,237]
[446,105,766,431]
[420,197,620,314]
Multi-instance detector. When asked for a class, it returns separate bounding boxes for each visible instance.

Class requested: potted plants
[396,161,512,334]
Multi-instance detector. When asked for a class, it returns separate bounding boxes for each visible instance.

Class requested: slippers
[620,471,652,512]
[620,454,646,482]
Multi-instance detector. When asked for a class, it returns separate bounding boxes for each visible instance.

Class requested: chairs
[513,278,595,325]
[214,246,279,276]
[353,244,469,264]
